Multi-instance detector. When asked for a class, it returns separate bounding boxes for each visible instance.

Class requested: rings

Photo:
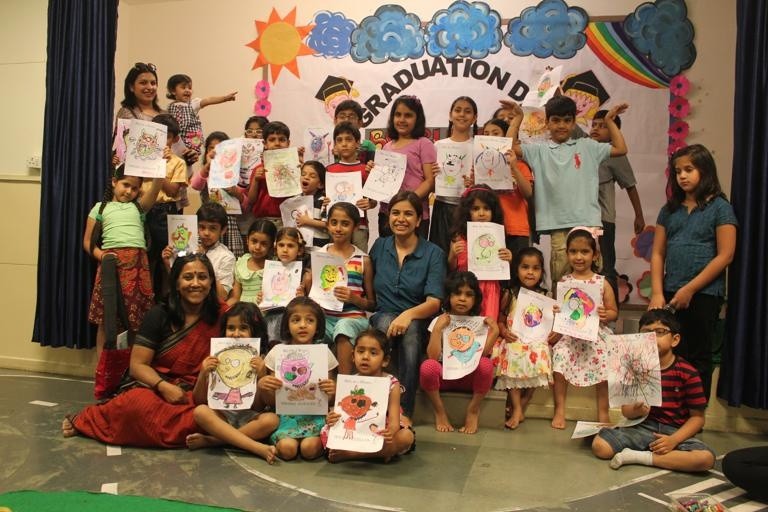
[397,332,401,334]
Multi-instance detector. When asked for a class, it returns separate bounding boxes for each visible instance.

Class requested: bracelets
[101,252,104,261]
[154,378,165,390]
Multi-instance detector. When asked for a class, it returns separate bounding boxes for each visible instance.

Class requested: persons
[248,121,304,233]
[226,219,279,318]
[546,66,646,334]
[299,100,363,196]
[647,144,738,433]
[321,329,416,464]
[63,252,230,449]
[367,191,444,429]
[256,227,305,350]
[136,114,188,303]
[322,121,377,254]
[551,226,618,430]
[432,96,478,259]
[365,95,437,238]
[489,247,554,429]
[166,74,238,207]
[448,184,512,387]
[237,117,271,237]
[303,202,375,374]
[112,62,199,165]
[297,161,330,268]
[584,309,715,472]
[470,119,534,288]
[84,146,172,364]
[494,108,521,144]
[500,95,630,300]
[257,296,336,460]
[186,301,279,465]
[419,271,499,434]
[190,131,247,260]
[162,202,236,301]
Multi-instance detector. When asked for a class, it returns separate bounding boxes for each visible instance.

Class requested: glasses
[244,129,265,137]
[336,115,358,121]
[179,248,206,258]
[639,327,674,337]
[135,62,155,70]
